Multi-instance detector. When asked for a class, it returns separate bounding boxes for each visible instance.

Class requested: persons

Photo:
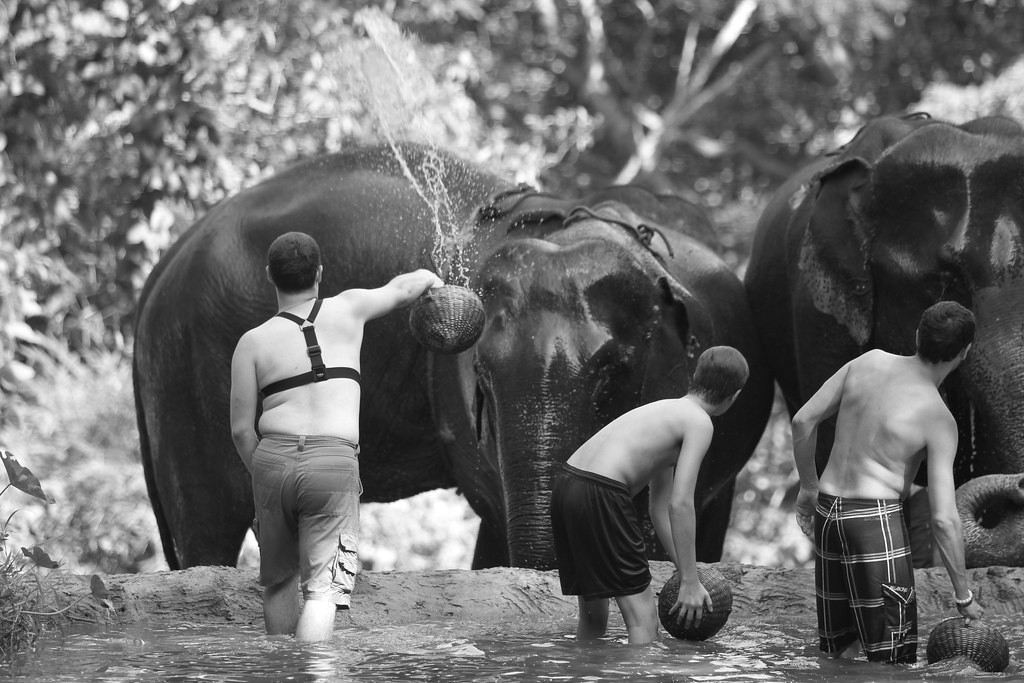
[788,299,988,668]
[552,345,749,648]
[231,232,446,645]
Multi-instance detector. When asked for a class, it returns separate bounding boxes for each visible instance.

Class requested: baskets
[926,616,1009,675]
[658,562,734,641]
[408,283,486,354]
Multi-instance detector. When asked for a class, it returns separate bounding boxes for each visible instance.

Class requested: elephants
[129,139,778,571]
[742,111,1024,567]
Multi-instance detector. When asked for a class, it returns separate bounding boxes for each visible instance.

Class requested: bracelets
[952,589,975,606]
